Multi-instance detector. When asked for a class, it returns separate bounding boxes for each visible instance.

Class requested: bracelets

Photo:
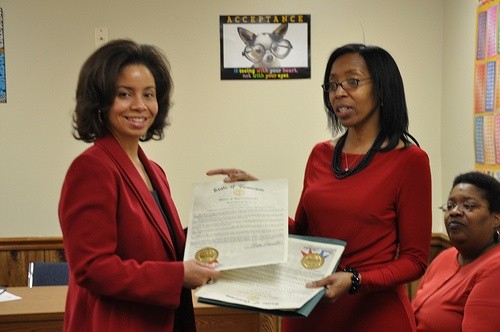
[344,265,361,297]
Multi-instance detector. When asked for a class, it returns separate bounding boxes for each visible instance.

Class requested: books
[199,233,347,318]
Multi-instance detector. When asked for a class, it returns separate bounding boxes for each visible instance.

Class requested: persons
[206,44,433,332]
[57,38,222,332]
[412,172,500,332]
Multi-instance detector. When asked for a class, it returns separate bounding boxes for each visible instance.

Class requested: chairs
[28,262,70,288]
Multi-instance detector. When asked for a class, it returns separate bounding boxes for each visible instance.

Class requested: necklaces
[330,128,387,180]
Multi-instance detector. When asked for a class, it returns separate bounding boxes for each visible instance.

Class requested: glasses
[439,200,490,214]
[241,39,293,63]
[321,76,373,92]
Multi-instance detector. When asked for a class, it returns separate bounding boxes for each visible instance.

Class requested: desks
[0,285,261,332]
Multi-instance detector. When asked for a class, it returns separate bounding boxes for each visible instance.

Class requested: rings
[207,275,214,284]
